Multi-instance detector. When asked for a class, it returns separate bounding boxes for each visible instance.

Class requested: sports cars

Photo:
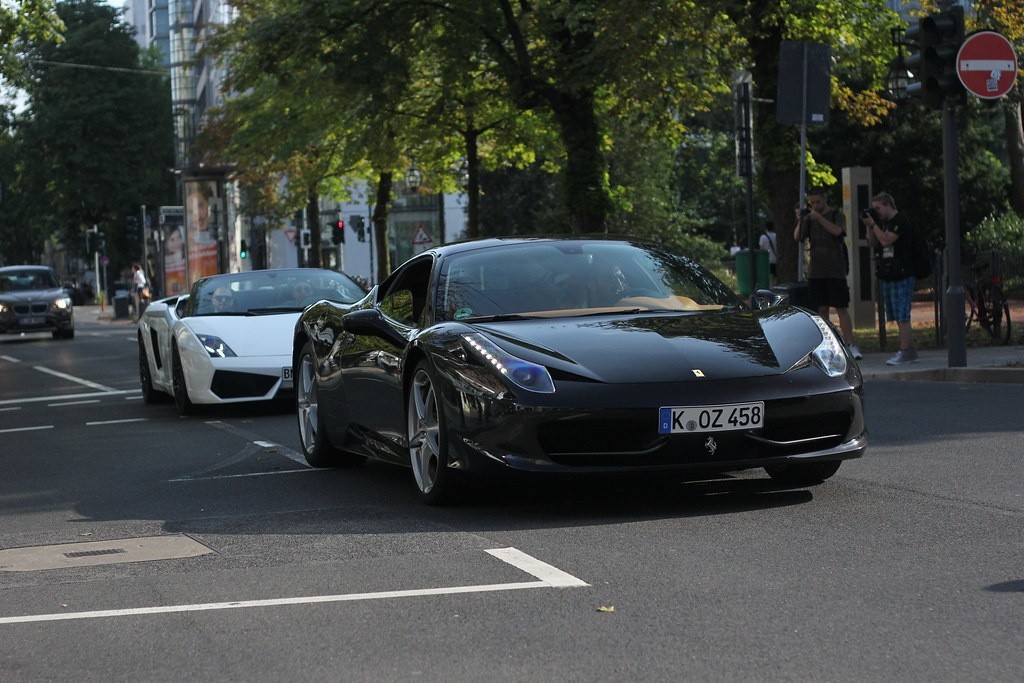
[135,267,368,414]
[291,232,871,507]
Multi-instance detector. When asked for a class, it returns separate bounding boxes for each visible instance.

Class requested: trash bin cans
[112,295,128,319]
[735,248,770,298]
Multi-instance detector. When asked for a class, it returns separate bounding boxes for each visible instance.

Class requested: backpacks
[890,216,930,280]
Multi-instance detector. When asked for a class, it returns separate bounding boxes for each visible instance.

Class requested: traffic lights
[901,18,927,97]
[920,6,964,94]
[336,220,345,245]
[241,241,247,260]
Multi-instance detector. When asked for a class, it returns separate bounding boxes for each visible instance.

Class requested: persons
[31,275,49,288]
[291,278,314,301]
[862,192,919,365]
[187,181,214,230]
[794,186,862,360]
[211,287,234,312]
[3,279,11,289]
[165,225,182,255]
[586,257,625,306]
[758,221,780,283]
[132,264,146,293]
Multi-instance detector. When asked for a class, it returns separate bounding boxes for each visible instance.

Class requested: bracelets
[871,222,875,229]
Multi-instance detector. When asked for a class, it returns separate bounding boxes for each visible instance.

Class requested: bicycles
[963,246,1012,344]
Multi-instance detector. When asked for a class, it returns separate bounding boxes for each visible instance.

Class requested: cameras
[800,203,813,215]
[862,207,877,220]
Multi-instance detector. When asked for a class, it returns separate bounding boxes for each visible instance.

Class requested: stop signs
[955,31,1016,99]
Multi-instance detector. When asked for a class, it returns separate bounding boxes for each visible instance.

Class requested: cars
[0,266,75,341]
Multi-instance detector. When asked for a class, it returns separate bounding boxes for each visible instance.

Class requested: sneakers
[887,350,920,365]
[849,344,863,359]
